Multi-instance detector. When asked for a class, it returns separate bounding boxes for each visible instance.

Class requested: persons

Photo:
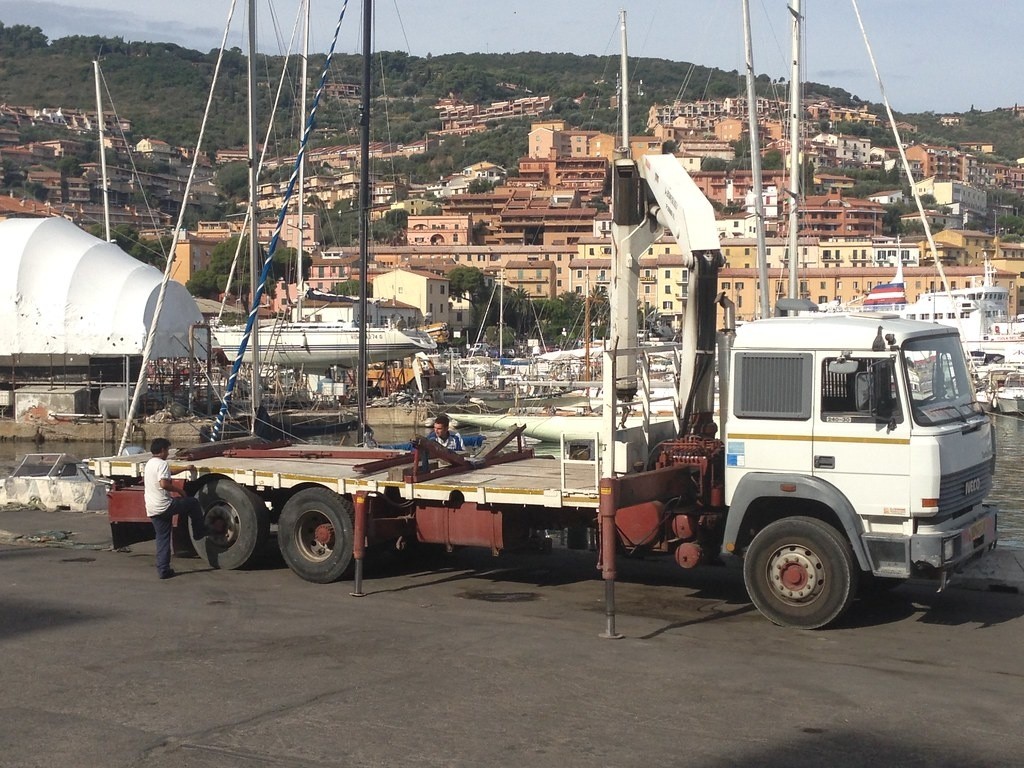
[144,438,211,579]
[376,416,487,451]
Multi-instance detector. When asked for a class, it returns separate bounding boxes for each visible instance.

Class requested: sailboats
[88,0,1023,445]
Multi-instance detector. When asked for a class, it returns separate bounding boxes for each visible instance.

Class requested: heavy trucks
[79,313,1001,640]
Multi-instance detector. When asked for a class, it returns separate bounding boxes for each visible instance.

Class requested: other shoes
[159,569,174,579]
[193,525,214,540]
